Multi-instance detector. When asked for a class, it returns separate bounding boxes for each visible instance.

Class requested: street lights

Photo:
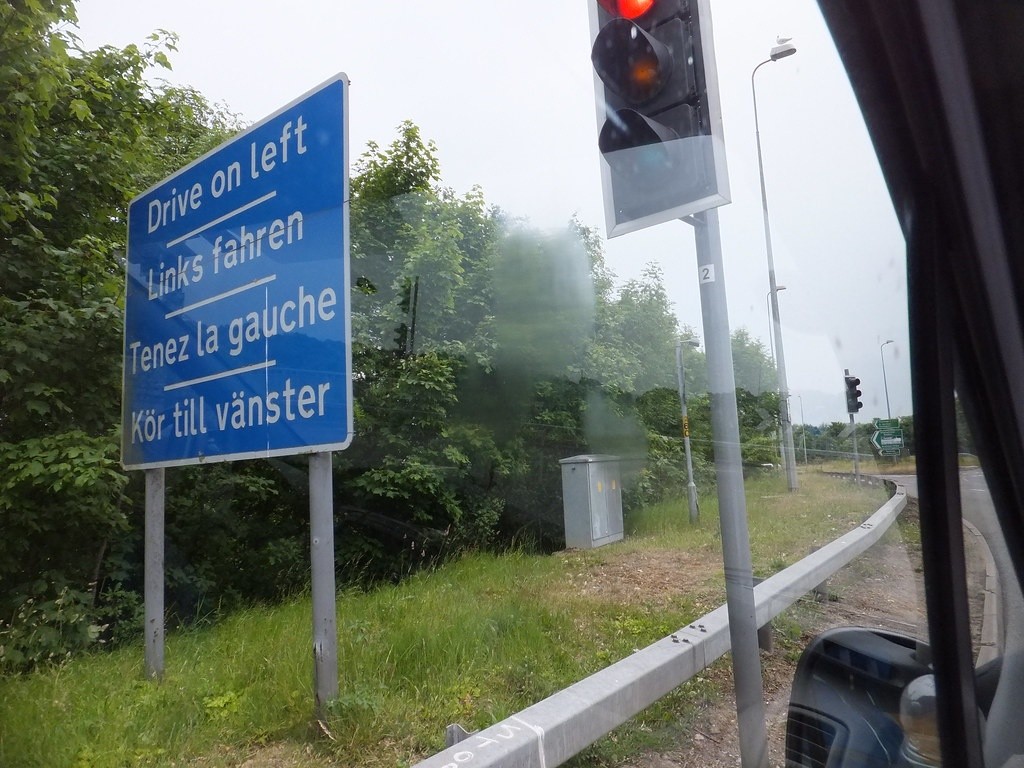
[797,395,808,464]
[766,285,788,472]
[750,43,802,493]
[880,340,898,466]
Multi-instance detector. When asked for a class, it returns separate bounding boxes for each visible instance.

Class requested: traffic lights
[845,376,863,412]
[589,0,732,241]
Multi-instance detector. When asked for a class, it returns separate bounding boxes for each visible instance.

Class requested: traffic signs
[871,429,905,449]
[873,418,901,430]
[878,448,901,457]
